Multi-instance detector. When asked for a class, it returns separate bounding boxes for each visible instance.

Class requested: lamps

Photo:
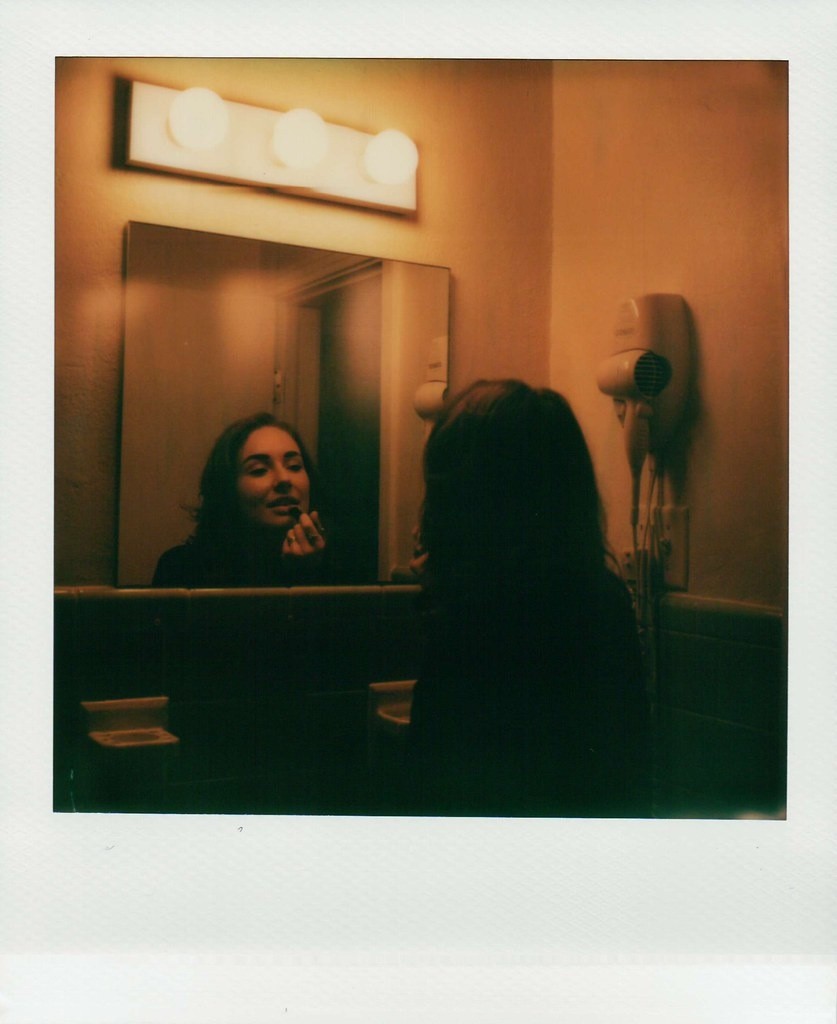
[126,79,417,214]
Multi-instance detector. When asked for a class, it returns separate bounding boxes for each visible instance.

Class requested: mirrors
[117,220,453,587]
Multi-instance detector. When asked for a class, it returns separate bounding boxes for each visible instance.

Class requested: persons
[151,411,351,587]
[403,379,654,818]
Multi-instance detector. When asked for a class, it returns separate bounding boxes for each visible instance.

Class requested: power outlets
[640,505,690,589]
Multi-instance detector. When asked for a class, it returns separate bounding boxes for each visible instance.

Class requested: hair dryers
[413,381,450,483]
[594,349,668,525]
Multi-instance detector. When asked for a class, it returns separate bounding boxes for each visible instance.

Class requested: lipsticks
[289,504,302,519]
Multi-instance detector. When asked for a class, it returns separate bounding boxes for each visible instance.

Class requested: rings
[306,533,318,540]
[287,537,295,542]
